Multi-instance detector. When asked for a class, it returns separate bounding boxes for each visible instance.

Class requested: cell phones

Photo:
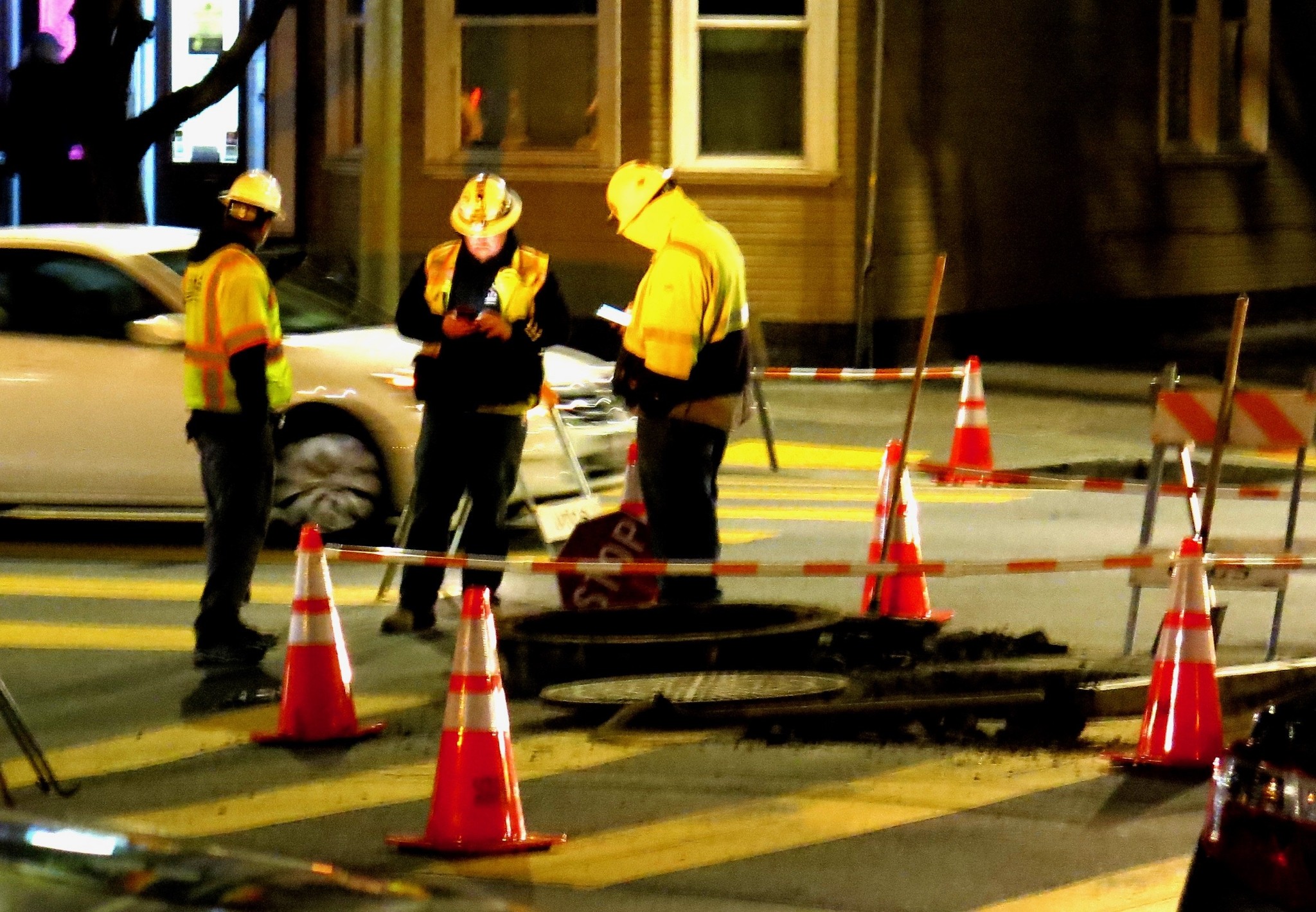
[457,308,480,321]
[593,304,629,328]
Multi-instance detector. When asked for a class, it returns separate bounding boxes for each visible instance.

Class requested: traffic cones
[249,522,384,745]
[922,353,998,485]
[1105,536,1230,776]
[386,586,567,856]
[865,438,955,628]
[617,442,648,525]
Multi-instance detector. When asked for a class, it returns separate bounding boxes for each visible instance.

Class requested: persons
[380,172,575,635]
[603,157,754,613]
[184,168,292,667]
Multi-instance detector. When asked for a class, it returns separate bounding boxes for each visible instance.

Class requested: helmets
[217,170,284,216]
[604,158,672,235]
[449,171,522,238]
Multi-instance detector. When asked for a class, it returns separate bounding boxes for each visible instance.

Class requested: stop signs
[554,511,660,610]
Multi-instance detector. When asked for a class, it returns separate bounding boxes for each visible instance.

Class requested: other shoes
[239,624,279,651]
[192,645,268,667]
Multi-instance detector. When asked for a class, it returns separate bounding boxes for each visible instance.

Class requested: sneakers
[380,606,435,632]
[490,594,501,606]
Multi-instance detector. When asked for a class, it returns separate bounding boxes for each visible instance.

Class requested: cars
[0,224,643,551]
